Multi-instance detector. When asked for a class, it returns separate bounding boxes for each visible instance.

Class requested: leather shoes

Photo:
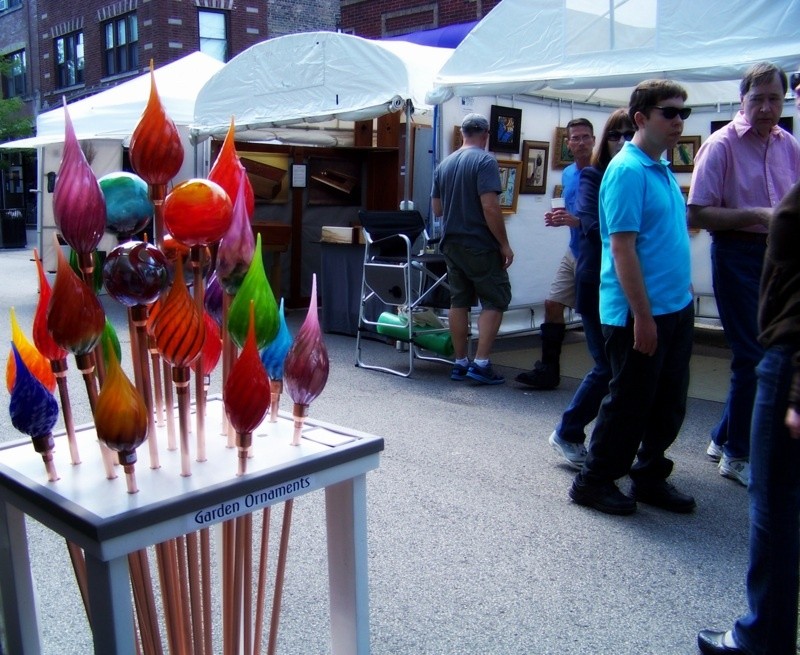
[698,627,743,654]
[569,473,637,518]
[630,476,696,513]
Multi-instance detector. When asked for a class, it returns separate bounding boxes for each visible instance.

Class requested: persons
[515,118,600,388]
[549,110,636,470]
[686,63,800,490]
[569,77,696,515]
[695,79,800,655]
[431,114,515,384]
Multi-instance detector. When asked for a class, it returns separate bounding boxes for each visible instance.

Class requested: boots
[514,322,566,387]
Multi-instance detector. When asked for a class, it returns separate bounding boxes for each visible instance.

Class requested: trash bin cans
[0,208,28,248]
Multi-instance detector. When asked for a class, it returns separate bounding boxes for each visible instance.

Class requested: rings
[561,217,563,221]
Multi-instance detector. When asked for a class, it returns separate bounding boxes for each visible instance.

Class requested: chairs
[354,209,473,378]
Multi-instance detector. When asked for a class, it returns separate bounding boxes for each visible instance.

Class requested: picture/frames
[495,159,523,215]
[552,185,565,211]
[666,135,701,173]
[518,139,551,195]
[452,124,464,155]
[552,126,576,171]
[488,104,523,154]
[680,185,700,234]
[710,116,794,137]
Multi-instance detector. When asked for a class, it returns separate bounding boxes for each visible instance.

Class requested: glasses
[607,130,637,142]
[652,105,692,119]
[569,135,594,142]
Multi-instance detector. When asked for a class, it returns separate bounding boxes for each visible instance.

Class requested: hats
[460,114,488,132]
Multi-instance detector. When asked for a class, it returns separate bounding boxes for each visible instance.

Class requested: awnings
[377,21,481,48]
[1,137,36,152]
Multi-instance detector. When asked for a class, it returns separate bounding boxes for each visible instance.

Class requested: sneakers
[451,362,470,381]
[547,430,587,472]
[707,440,722,461]
[720,450,752,487]
[466,359,505,385]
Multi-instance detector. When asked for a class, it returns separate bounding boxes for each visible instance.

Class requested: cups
[551,198,566,213]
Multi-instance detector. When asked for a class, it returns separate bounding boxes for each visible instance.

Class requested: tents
[36,52,230,275]
[189,31,456,304]
[422,0,800,240]
